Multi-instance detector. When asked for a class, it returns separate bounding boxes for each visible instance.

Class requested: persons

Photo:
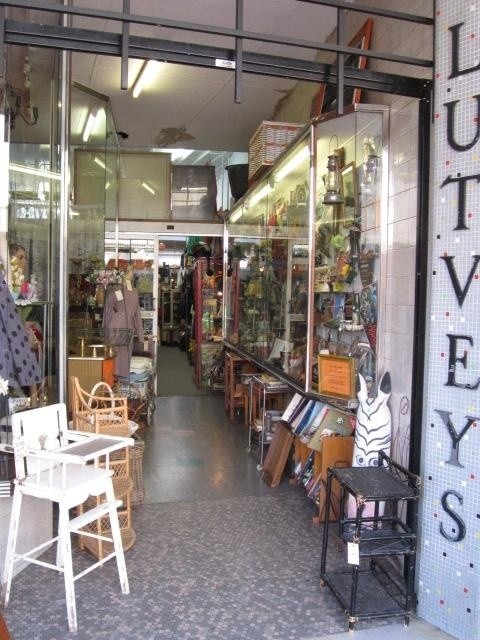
[9,244,29,274]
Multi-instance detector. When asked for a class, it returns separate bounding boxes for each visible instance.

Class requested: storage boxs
[259,419,296,490]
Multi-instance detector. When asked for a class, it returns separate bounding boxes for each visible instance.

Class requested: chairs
[2,402,131,634]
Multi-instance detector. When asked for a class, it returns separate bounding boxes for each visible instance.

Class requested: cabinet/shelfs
[319,450,421,636]
[287,393,357,527]
[248,381,287,426]
[225,102,389,396]
[244,372,295,472]
[191,257,227,391]
[66,231,159,423]
[66,354,118,411]
[224,350,252,425]
[160,266,188,346]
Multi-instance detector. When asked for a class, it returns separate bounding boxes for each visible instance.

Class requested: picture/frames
[313,17,373,115]
[318,354,355,401]
[316,352,357,404]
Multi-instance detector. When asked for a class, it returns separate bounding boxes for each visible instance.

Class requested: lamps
[130,58,157,98]
[141,181,156,196]
[8,162,62,183]
[81,107,98,143]
[179,186,207,192]
[93,156,113,174]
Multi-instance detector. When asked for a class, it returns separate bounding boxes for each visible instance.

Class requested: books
[281,392,356,453]
